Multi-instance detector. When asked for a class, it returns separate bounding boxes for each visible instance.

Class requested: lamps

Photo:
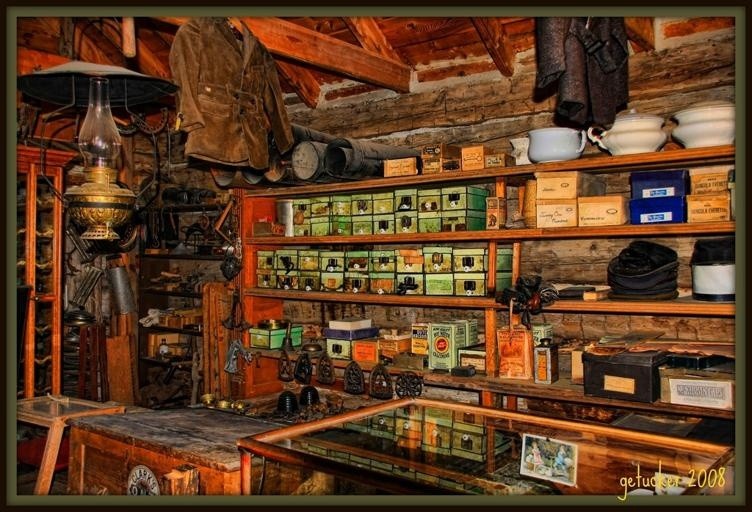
[17,59,179,242]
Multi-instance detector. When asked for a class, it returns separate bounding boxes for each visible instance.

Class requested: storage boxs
[422,424,452,456]
[426,273,453,295]
[344,273,370,293]
[394,189,417,211]
[453,248,513,272]
[367,414,393,439]
[395,211,417,234]
[327,450,350,465]
[659,365,735,412]
[370,459,393,476]
[368,251,396,273]
[451,431,512,463]
[628,170,691,199]
[277,270,299,289]
[422,247,453,273]
[687,192,735,223]
[534,171,602,199]
[429,324,466,371]
[248,325,303,349]
[582,351,671,405]
[332,196,352,215]
[454,273,513,295]
[352,215,373,235]
[393,464,416,479]
[352,195,373,215]
[331,215,352,236]
[395,418,422,451]
[319,251,345,272]
[353,338,412,370]
[424,407,453,427]
[257,250,278,269]
[442,319,479,347]
[293,199,312,218]
[453,409,489,434]
[395,250,423,273]
[345,251,369,273]
[321,273,344,293]
[276,250,298,270]
[536,200,578,228]
[369,273,396,294]
[312,217,332,236]
[578,196,631,227]
[373,192,395,214]
[297,250,319,271]
[396,273,425,296]
[690,164,735,195]
[256,270,277,290]
[373,214,395,234]
[442,210,486,232]
[63,406,293,496]
[236,395,735,495]
[310,196,333,217]
[442,187,490,211]
[299,271,320,291]
[348,454,371,467]
[294,219,311,236]
[324,328,378,360]
[418,189,442,212]
[630,196,688,225]
[440,479,463,492]
[307,444,327,458]
[418,212,442,234]
[417,472,442,486]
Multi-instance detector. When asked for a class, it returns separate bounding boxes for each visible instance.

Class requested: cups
[276,392,297,411]
[301,387,319,402]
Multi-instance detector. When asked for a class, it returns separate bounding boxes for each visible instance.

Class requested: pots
[672,100,738,149]
[527,127,587,162]
[588,108,667,155]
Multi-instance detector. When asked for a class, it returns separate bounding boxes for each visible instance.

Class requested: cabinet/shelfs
[16,141,78,438]
[140,200,236,409]
[231,145,736,429]
